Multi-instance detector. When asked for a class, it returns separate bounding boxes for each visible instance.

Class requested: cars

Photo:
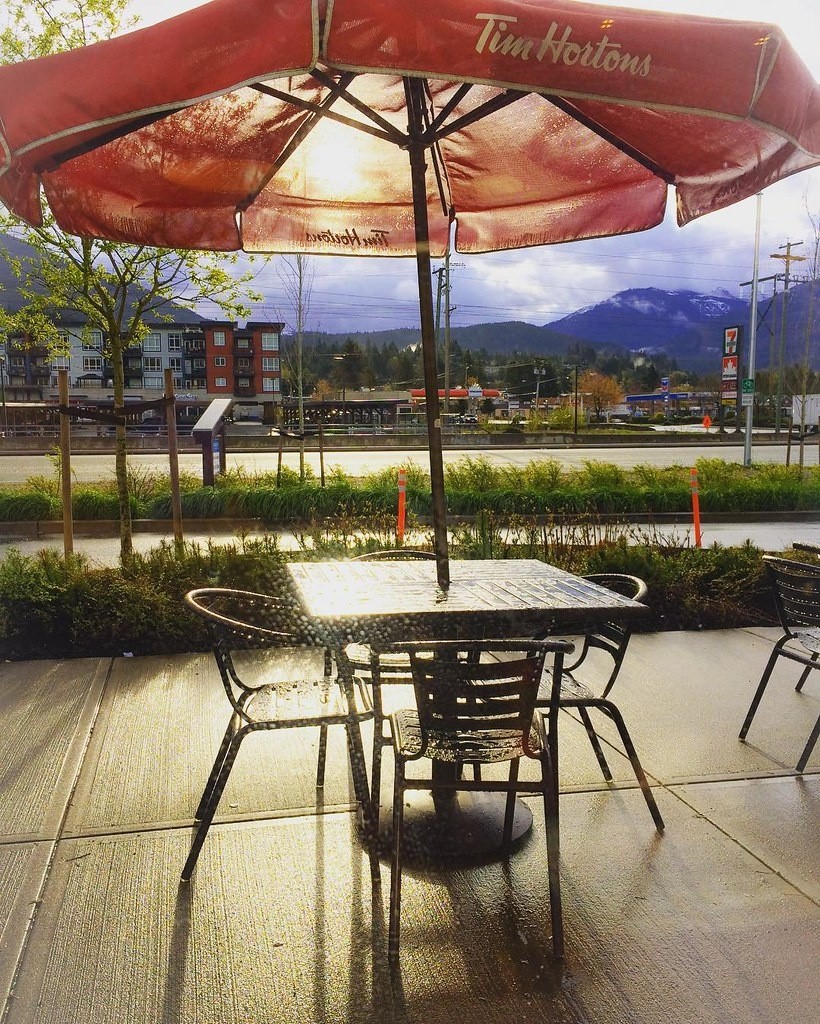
[328,412,355,423]
[274,418,312,433]
[461,414,477,423]
[101,425,145,437]
[137,417,166,426]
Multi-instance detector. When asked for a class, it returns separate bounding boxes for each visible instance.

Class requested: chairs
[178,588,381,884]
[738,553,820,775]
[369,638,575,961]
[315,549,451,788]
[456,573,664,831]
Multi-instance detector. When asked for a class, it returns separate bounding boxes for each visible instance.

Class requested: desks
[287,559,647,858]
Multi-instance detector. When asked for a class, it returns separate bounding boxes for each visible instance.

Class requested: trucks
[792,394,820,433]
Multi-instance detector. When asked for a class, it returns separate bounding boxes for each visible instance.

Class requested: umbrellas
[0,1,820,771]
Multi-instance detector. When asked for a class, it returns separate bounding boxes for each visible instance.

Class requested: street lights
[0,356,8,436]
[271,377,275,409]
[575,365,581,434]
[536,377,570,406]
[334,357,346,423]
